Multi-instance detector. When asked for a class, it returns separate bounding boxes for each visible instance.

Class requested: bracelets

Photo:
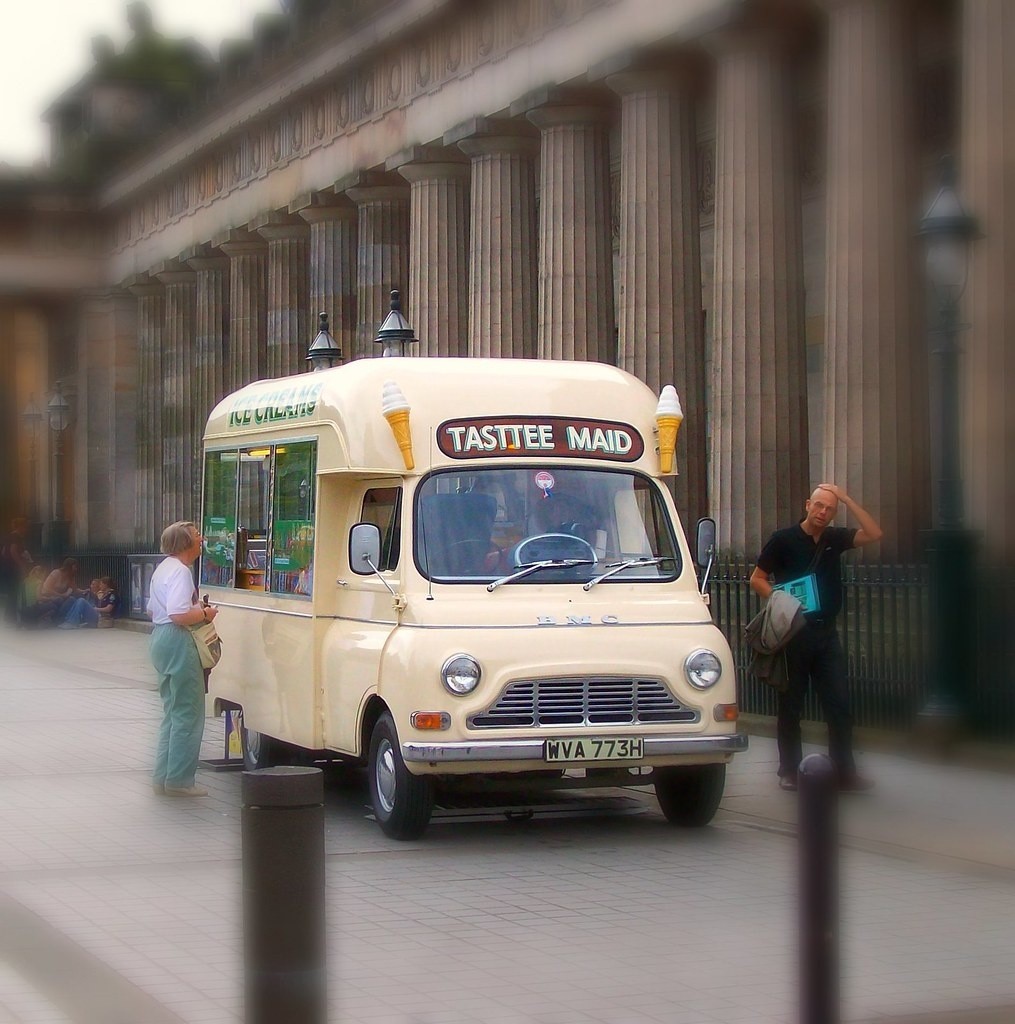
[202,609,207,621]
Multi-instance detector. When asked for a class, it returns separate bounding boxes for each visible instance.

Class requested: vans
[195,358,745,840]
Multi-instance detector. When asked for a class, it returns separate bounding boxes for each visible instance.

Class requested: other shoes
[841,767,873,789]
[779,770,797,791]
[58,622,80,630]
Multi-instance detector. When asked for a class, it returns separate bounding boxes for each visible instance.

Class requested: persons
[482,477,590,574]
[751,483,881,791]
[2,518,117,629]
[146,522,219,796]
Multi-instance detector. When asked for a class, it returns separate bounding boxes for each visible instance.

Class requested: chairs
[525,493,601,562]
[418,494,498,577]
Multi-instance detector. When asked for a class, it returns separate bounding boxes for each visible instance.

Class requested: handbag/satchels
[97,617,113,628]
[744,608,778,655]
[191,621,223,669]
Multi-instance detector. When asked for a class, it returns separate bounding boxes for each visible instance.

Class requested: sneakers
[152,784,164,795]
[164,783,208,797]
